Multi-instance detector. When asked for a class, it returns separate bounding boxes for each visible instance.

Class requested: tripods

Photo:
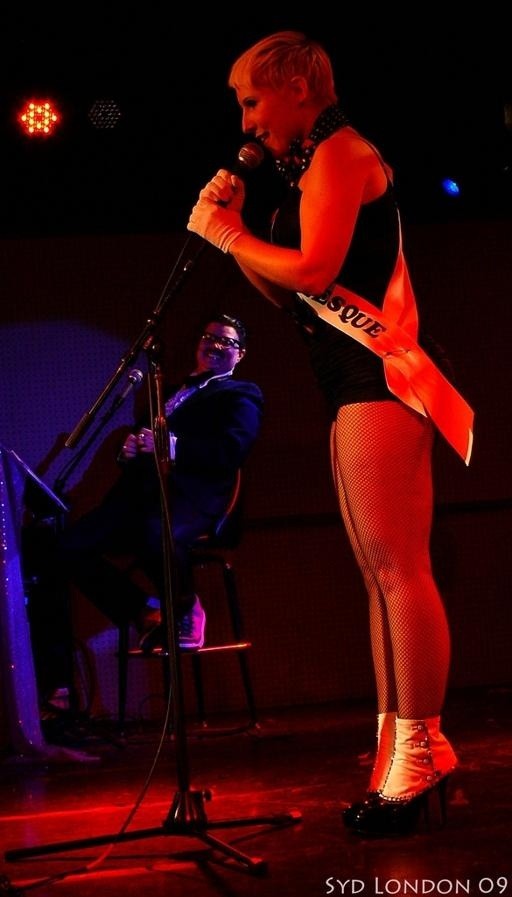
[0,230,307,871]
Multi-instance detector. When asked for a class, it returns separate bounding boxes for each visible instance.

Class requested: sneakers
[137,610,160,651]
[177,595,207,648]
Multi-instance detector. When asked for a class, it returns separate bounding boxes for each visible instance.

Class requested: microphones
[114,367,144,407]
[223,142,265,178]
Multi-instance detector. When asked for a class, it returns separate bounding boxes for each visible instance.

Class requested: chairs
[114,464,259,733]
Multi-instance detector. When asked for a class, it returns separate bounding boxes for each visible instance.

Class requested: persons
[185,31,475,839]
[69,311,265,655]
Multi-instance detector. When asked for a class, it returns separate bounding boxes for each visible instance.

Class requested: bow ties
[184,371,213,387]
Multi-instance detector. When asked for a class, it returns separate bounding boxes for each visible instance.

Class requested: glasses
[202,333,242,349]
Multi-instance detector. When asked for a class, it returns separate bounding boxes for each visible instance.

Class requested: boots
[341,711,460,840]
